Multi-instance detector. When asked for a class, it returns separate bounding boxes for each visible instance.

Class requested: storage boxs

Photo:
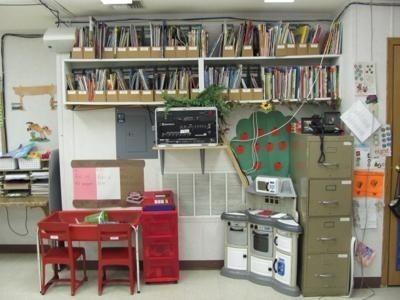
[220,210,303,296]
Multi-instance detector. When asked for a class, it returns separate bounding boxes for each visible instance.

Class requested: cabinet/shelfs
[289,131,354,296]
[61,54,340,107]
[0,149,63,272]
[141,189,180,283]
[35,211,140,294]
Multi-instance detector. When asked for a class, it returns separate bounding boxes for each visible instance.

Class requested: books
[0,152,52,200]
[65,13,344,102]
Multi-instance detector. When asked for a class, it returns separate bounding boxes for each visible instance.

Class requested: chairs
[38,222,89,297]
[97,223,136,295]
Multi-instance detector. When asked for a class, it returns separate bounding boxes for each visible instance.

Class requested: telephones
[309,120,340,136]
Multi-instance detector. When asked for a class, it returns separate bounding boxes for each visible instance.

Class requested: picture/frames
[301,117,315,134]
